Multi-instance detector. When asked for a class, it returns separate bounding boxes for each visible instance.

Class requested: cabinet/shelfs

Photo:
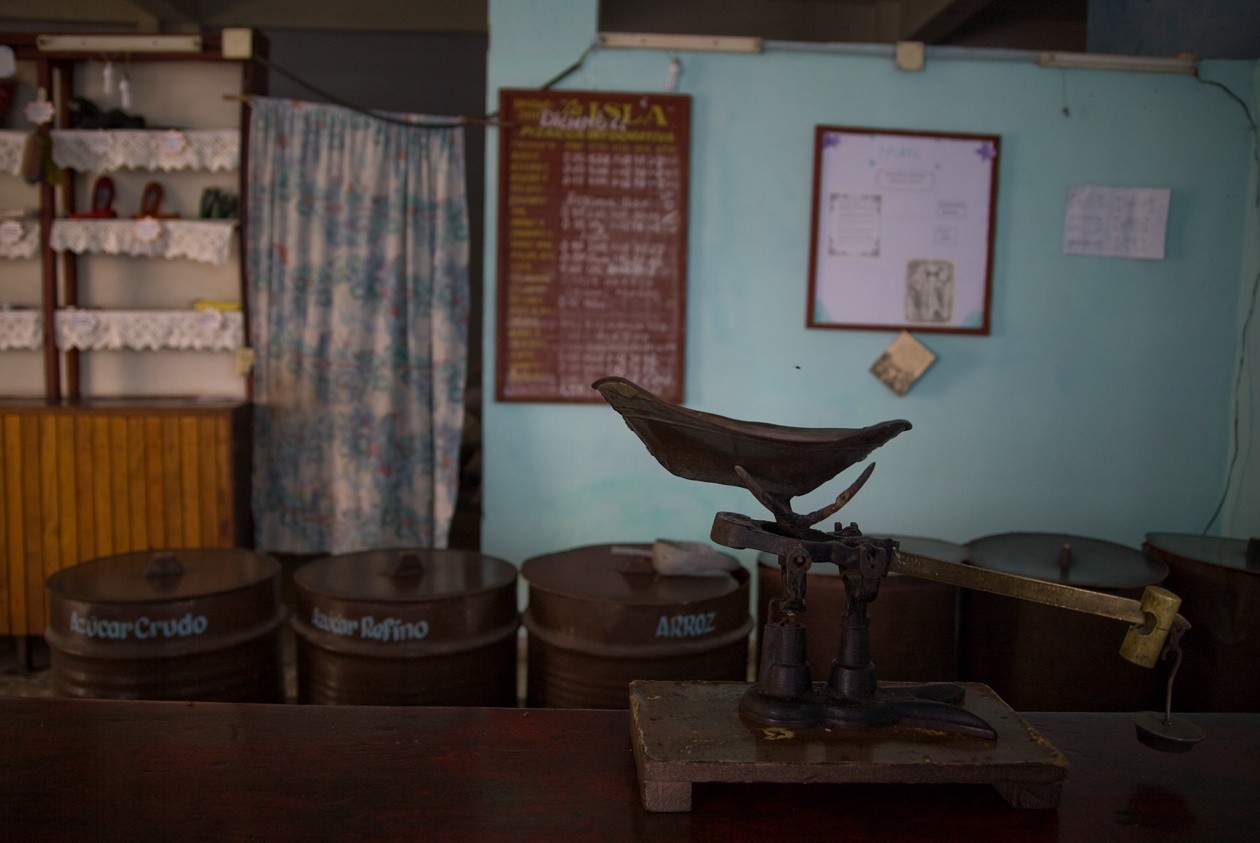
[0,24,269,403]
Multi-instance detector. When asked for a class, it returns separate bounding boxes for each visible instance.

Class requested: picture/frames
[807,121,1003,337]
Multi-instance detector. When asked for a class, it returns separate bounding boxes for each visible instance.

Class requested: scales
[590,375,1208,753]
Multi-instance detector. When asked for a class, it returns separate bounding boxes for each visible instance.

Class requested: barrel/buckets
[965,530,1171,712]
[1142,534,1260,711]
[758,532,966,686]
[289,548,525,708]
[522,542,757,711]
[41,548,285,704]
